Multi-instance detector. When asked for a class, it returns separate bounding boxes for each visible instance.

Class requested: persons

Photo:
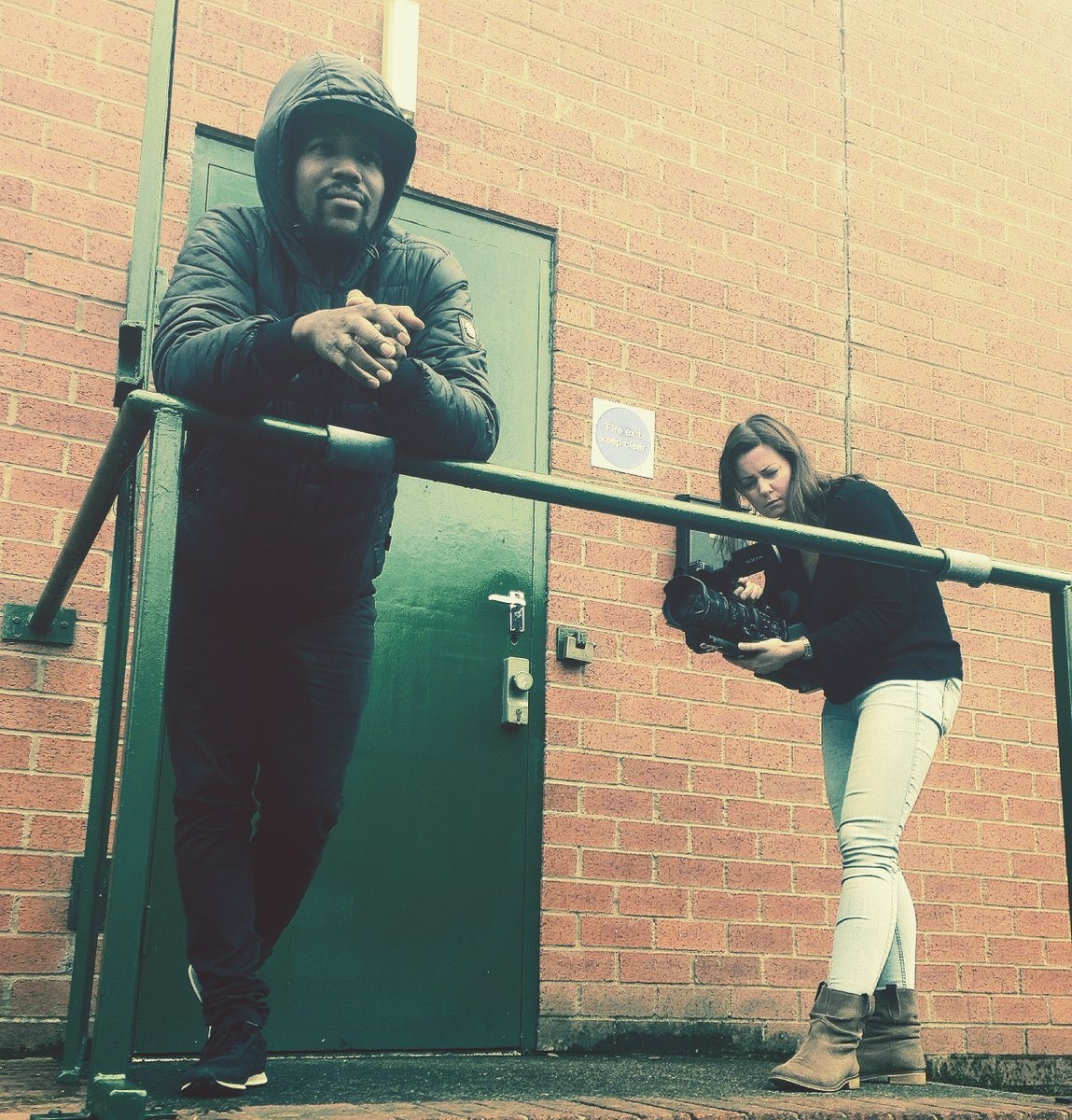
[716,413,965,1092]
[151,51,500,1099]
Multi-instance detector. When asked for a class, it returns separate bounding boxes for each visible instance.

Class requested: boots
[857,984,927,1085]
[769,981,875,1093]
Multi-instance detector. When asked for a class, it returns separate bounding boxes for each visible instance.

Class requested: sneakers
[181,1022,268,1097]
[188,965,206,1004]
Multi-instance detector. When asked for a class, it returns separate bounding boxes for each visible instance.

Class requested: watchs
[800,635,815,662]
